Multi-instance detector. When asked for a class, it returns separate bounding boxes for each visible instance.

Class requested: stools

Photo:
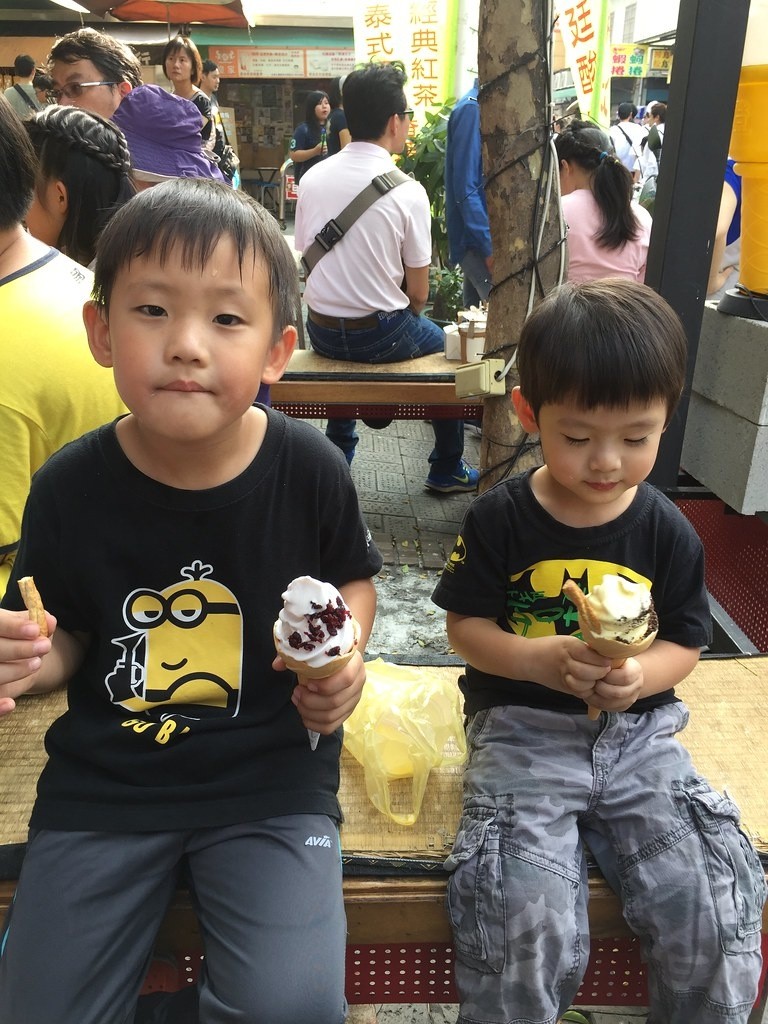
[241,178,259,201]
[259,182,280,214]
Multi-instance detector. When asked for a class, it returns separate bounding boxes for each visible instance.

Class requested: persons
[437,273,764,1023]
[0,100,135,608]
[4,33,247,272]
[290,89,332,183]
[545,94,752,294]
[324,75,356,159]
[0,178,376,1024]
[445,59,520,308]
[295,65,488,497]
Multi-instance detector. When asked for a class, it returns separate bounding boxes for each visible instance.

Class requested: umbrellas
[107,0,247,51]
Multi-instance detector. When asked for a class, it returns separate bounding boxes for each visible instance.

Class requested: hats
[108,83,223,179]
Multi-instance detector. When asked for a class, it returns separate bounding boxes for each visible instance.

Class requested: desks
[240,166,280,208]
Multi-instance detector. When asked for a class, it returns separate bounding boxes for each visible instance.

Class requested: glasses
[397,107,414,121]
[45,81,119,104]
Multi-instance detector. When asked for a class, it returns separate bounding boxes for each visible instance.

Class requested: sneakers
[426,461,481,493]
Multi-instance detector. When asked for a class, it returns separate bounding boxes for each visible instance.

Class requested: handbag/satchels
[220,144,241,181]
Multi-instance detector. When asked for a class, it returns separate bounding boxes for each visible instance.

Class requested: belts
[308,309,380,330]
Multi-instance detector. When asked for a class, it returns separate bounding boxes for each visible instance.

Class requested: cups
[458,319,486,363]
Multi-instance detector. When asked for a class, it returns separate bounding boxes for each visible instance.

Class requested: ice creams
[562,574,659,722]
[272,576,361,754]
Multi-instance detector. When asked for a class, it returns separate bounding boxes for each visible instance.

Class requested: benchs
[0,650,768,943]
[263,346,486,422]
